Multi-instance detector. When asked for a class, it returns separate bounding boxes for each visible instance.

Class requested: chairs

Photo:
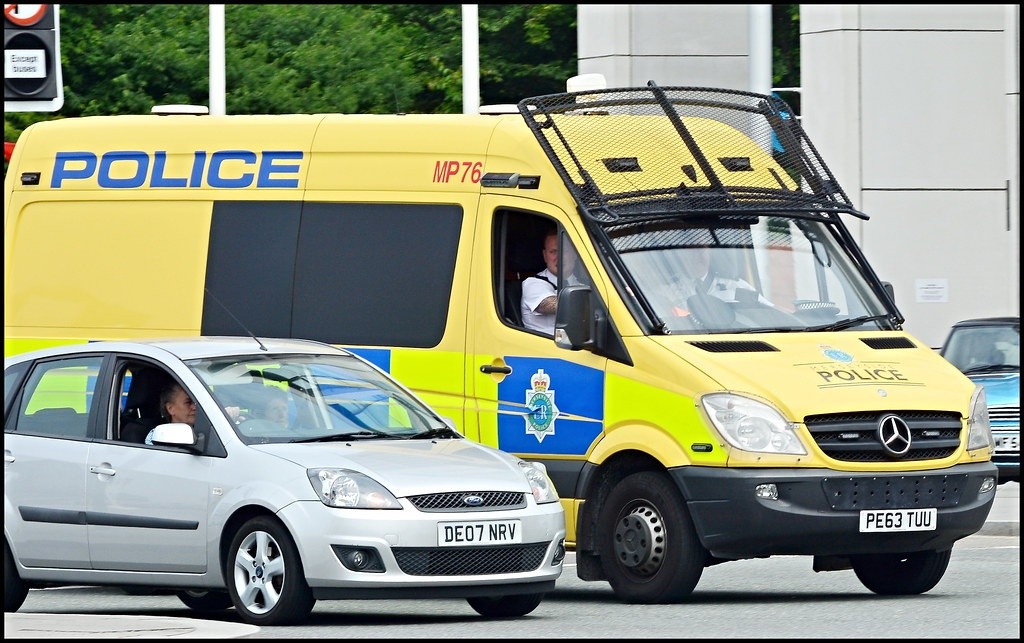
[32,408,80,440]
[120,379,173,444]
[504,243,551,335]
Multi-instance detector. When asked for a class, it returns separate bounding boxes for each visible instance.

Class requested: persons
[145,383,244,451]
[254,386,291,432]
[666,227,775,316]
[521,231,611,337]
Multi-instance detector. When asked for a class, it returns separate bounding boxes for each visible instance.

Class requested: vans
[5,74,999,604]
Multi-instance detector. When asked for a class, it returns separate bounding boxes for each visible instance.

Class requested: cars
[4,337,566,627]
[939,316,1020,485]
